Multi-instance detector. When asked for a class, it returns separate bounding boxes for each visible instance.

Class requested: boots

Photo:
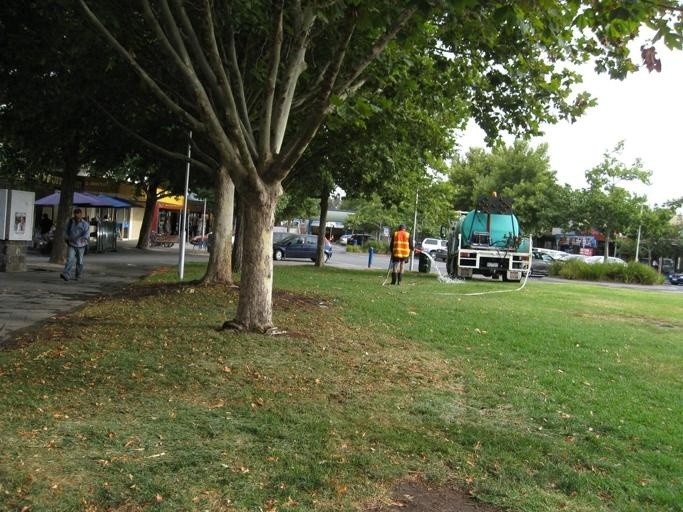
[398,273,403,284]
[390,272,397,284]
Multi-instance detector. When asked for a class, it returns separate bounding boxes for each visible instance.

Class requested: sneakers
[59,273,68,281]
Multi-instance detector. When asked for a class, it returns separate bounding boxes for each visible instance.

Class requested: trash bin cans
[347,238,357,245]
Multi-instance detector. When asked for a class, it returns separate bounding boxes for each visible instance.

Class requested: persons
[161,217,212,247]
[38,210,108,241]
[14,212,26,233]
[59,208,90,282]
[388,223,423,286]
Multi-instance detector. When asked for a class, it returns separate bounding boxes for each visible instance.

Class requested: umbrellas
[75,192,116,221]
[310,220,344,240]
[34,188,100,224]
[97,192,145,248]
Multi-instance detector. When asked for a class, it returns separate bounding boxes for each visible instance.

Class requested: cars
[340,233,375,246]
[189,232,215,247]
[667,273,683,285]
[272,234,333,263]
[521,247,629,278]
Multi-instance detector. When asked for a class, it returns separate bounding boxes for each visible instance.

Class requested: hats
[399,224,407,228]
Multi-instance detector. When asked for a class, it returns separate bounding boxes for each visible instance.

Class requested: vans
[661,257,674,272]
[420,236,451,260]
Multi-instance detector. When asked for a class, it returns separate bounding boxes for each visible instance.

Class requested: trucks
[441,191,531,282]
